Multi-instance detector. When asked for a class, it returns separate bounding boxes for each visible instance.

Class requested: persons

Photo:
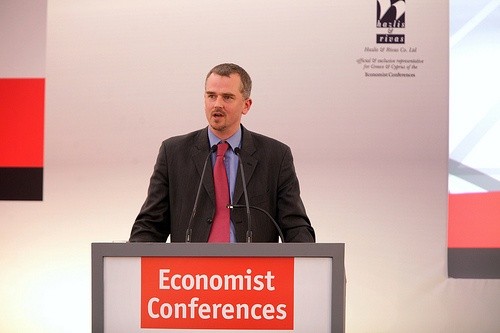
[129,64,316,243]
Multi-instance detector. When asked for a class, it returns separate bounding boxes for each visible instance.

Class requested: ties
[206,141,230,243]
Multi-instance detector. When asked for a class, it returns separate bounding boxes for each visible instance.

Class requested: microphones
[226,205,285,243]
[234,147,252,243]
[186,144,218,242]
